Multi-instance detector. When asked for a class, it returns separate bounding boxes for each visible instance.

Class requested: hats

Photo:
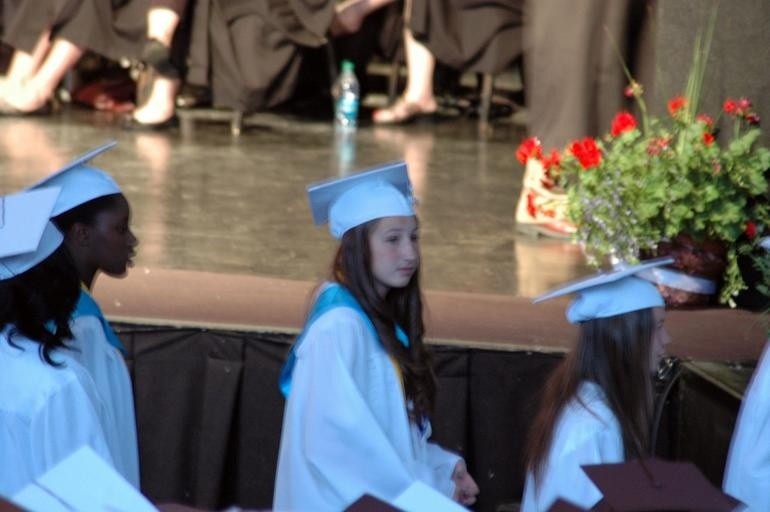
[308,160,419,238]
[28,141,125,216]
[533,257,667,326]
[0,187,66,281]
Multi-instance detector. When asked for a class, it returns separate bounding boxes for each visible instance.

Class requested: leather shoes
[1,98,60,117]
[140,37,179,78]
[117,111,180,129]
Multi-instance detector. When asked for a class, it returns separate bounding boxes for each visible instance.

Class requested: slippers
[374,96,437,126]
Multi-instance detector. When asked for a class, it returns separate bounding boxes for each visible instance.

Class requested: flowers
[514,8,768,309]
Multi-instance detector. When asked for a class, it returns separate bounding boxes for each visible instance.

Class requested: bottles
[332,59,361,136]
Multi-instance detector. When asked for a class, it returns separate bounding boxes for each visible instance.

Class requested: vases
[648,235,725,309]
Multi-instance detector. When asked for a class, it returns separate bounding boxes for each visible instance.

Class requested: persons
[0,2,146,116]
[372,2,524,128]
[131,1,334,130]
[516,306,673,511]
[0,236,116,500]
[271,215,480,512]
[41,194,144,502]
